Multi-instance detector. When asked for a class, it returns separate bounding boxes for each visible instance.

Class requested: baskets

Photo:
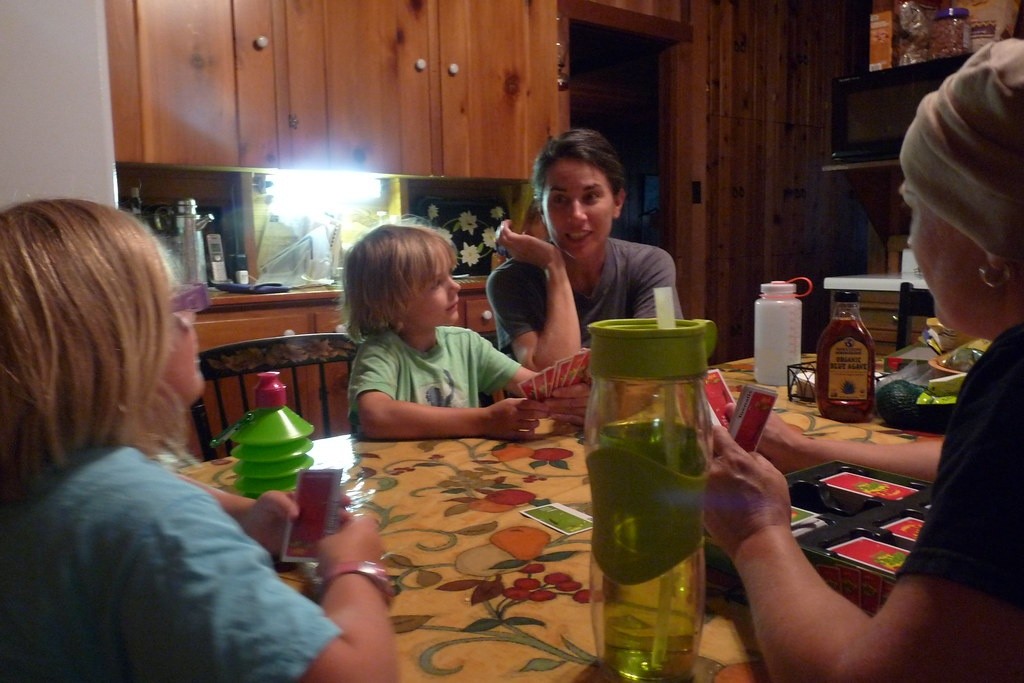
[787,360,890,404]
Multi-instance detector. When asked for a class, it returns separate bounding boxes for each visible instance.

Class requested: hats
[899,40,1024,264]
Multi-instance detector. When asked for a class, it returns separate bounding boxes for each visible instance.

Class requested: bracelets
[321,560,401,598]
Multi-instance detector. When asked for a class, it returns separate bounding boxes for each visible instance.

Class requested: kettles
[154,198,215,289]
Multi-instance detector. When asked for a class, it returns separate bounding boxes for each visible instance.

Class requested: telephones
[207,233,230,285]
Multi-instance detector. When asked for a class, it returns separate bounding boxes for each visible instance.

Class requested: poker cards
[880,517,925,540]
[826,537,911,576]
[819,470,919,499]
[699,369,779,455]
[788,506,818,526]
[521,502,594,534]
[517,347,592,402]
[280,468,344,562]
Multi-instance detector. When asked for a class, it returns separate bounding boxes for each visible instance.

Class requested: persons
[342,224,591,442]
[485,128,684,373]
[703,38,1024,683]
[0,198,402,683]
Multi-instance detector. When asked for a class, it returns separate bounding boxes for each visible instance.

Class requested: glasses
[163,283,209,313]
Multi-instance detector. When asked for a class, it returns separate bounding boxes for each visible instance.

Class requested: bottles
[752,276,813,387]
[813,290,876,424]
[229,369,315,572]
[581,317,717,683]
[928,8,971,60]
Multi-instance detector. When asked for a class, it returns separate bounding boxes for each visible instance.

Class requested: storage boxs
[869,10,900,72]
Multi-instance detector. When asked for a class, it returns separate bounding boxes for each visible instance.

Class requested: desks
[175,354,943,683]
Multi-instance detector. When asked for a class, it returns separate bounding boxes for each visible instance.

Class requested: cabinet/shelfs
[189,278,496,460]
[104,0,557,180]
[832,289,930,357]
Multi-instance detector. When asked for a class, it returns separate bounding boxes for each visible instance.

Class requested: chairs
[191,332,358,460]
[896,282,936,350]
